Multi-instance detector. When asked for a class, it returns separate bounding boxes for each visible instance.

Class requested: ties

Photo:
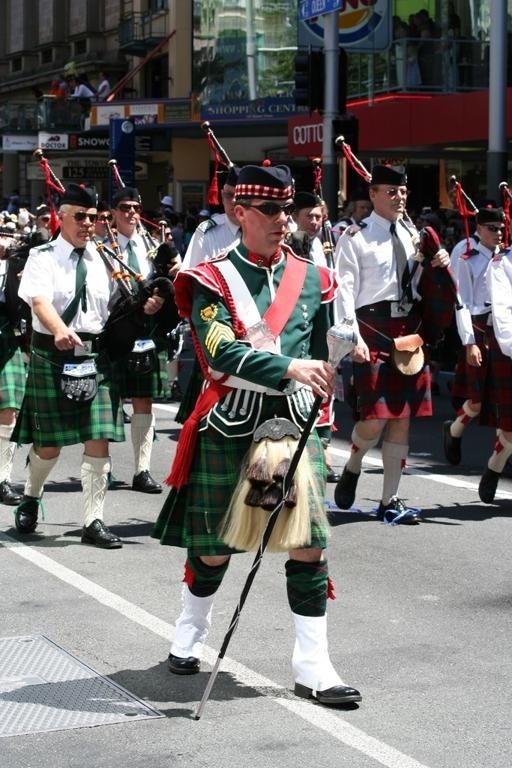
[61,246,88,326]
[388,223,416,303]
[125,239,140,294]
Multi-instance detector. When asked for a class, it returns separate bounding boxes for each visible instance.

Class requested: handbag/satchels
[59,360,98,402]
[392,334,426,376]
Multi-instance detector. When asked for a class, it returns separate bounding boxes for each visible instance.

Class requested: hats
[289,190,326,210]
[199,209,210,217]
[109,187,141,206]
[420,213,442,231]
[225,164,295,201]
[352,189,370,201]
[161,196,173,207]
[57,184,99,208]
[370,163,409,186]
[473,206,508,223]
[37,207,52,217]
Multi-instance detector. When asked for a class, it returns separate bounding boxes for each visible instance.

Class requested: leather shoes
[479,457,503,503]
[325,461,341,484]
[442,420,463,465]
[14,495,39,532]
[290,682,362,706]
[333,463,361,509]
[375,494,423,522]
[80,519,123,549]
[0,479,22,505]
[167,652,216,675]
[131,470,163,494]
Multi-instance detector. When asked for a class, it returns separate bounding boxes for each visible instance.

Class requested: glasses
[66,210,98,224]
[483,223,506,233]
[98,214,113,222]
[247,201,296,216]
[116,203,143,214]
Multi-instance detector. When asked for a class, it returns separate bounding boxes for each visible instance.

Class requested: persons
[1,163,511,547]
[149,165,362,705]
[62,66,115,104]
